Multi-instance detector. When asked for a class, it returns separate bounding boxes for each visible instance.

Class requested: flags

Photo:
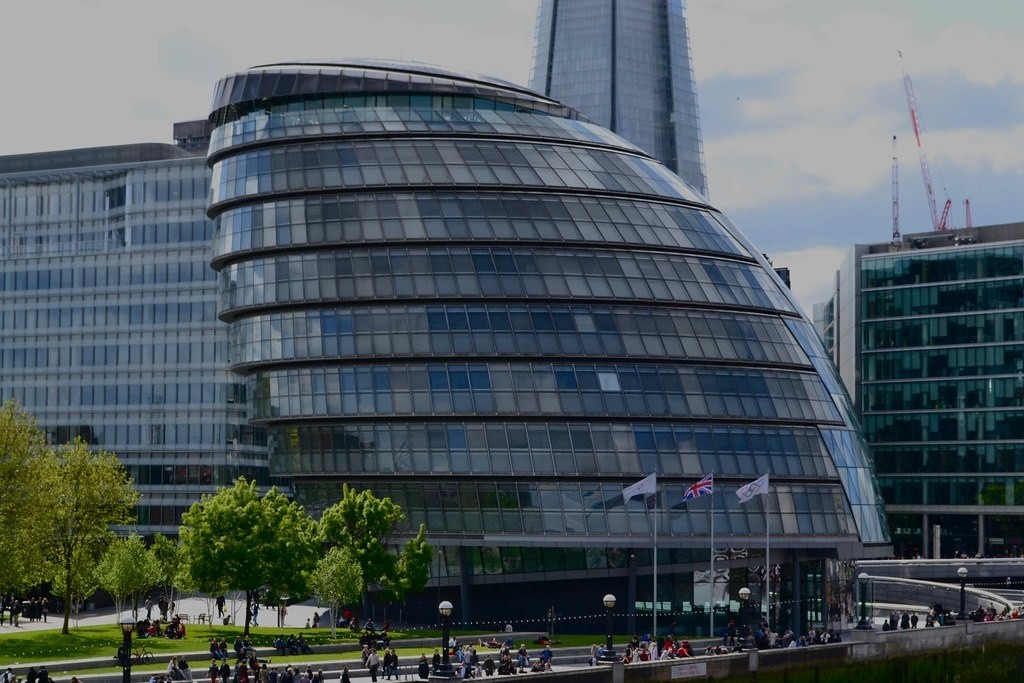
[622,472,656,504]
[734,473,769,504]
[682,472,714,502]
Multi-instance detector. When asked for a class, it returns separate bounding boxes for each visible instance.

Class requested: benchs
[193,613,205,624]
[179,613,190,624]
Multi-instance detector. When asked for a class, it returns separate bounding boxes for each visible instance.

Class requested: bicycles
[130,642,156,666]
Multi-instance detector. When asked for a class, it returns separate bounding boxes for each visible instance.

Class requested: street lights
[955,566,970,621]
[598,592,617,659]
[735,585,754,639]
[117,615,139,683]
[854,571,874,629]
[432,598,456,680]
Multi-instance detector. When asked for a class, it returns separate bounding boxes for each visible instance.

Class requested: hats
[682,640,688,644]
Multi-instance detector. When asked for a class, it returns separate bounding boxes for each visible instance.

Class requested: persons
[339,666,352,683]
[0,665,83,683]
[337,607,392,632]
[954,548,1024,559]
[304,612,321,630]
[587,633,694,666]
[881,611,959,631]
[135,591,186,640]
[223,615,232,626]
[704,618,842,656]
[359,631,399,683]
[0,596,22,627]
[280,603,289,628]
[147,655,194,683]
[968,601,1024,622]
[504,622,513,632]
[246,597,262,627]
[29,596,49,623]
[214,592,228,618]
[887,552,927,560]
[206,631,324,683]
[115,641,126,668]
[416,633,561,679]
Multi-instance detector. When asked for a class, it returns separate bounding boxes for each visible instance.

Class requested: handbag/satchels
[525,659,529,666]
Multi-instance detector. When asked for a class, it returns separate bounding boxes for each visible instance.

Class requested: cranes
[891,50,972,239]
